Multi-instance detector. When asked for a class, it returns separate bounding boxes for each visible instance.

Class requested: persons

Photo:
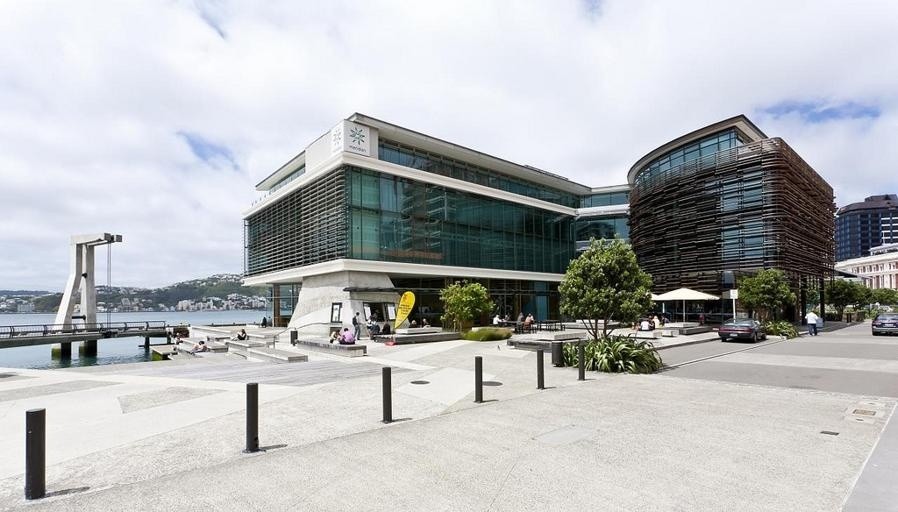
[518,313,534,325]
[423,319,429,327]
[234,329,246,341]
[409,320,417,328]
[167,331,185,345]
[492,315,511,324]
[262,317,272,327]
[193,341,207,352]
[805,310,819,336]
[329,328,355,345]
[353,312,390,340]
[631,312,669,332]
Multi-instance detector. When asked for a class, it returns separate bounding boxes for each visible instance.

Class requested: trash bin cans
[291,330,298,345]
[846,313,852,323]
[551,340,565,367]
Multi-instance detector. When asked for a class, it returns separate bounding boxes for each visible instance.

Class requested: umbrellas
[651,288,721,322]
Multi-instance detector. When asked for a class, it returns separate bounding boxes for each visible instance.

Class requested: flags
[394,291,415,328]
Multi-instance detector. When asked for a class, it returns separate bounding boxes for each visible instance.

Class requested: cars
[871,312,897,335]
[718,317,766,343]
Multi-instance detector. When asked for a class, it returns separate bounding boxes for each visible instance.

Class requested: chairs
[489,320,566,333]
